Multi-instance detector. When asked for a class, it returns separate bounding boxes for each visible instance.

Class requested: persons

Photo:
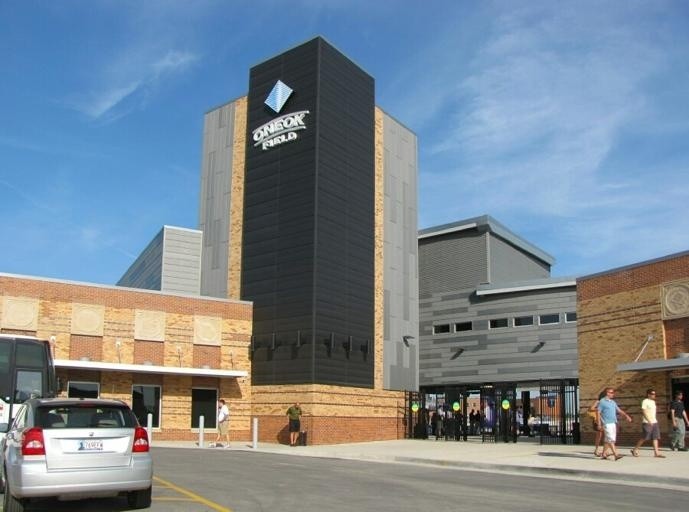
[594,387,633,461]
[208,399,231,448]
[285,402,302,447]
[670,389,689,452]
[431,405,480,438]
[631,388,666,457]
[587,392,615,457]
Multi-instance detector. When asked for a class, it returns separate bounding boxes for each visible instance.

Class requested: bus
[0,334,56,443]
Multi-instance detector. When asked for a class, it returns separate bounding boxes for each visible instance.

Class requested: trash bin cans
[299,431,307,446]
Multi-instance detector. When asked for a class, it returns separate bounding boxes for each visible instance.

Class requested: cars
[0,397,153,512]
